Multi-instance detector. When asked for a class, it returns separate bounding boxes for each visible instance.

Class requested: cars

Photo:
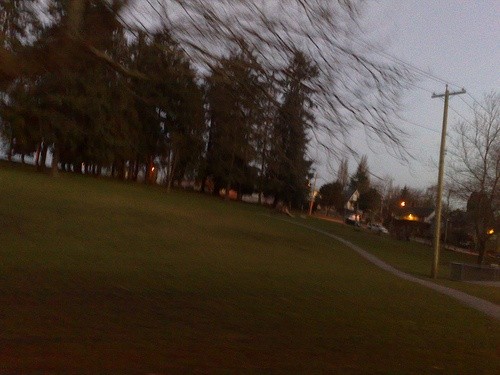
[367,222,388,234]
